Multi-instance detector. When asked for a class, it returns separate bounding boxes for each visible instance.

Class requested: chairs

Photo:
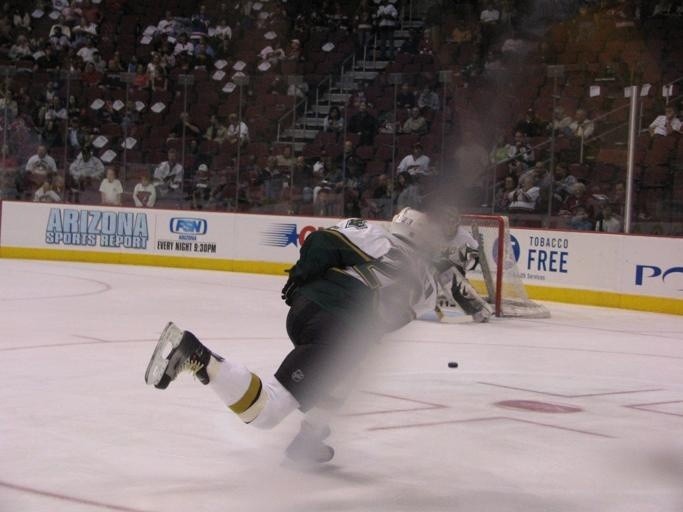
[0,0,682,237]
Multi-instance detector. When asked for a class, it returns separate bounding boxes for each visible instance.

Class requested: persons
[2,0,399,207]
[390,203,493,323]
[393,0,682,233]
[154,206,443,466]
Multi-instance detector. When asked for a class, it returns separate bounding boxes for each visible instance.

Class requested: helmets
[390,205,429,245]
[441,205,462,228]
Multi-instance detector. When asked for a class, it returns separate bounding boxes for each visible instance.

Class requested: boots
[153,329,227,390]
[284,415,336,464]
